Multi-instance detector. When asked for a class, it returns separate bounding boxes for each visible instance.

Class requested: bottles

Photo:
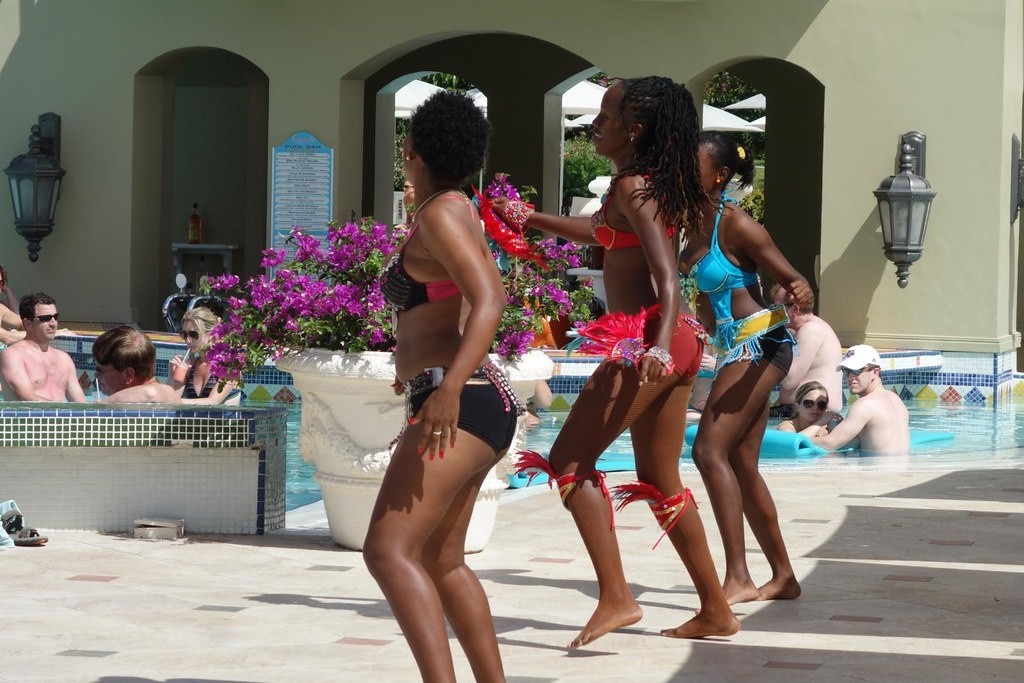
[188,204,203,244]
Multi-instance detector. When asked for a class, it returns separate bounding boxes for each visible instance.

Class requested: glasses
[802,399,827,411]
[771,303,793,310]
[95,366,115,373]
[23,313,60,322]
[844,367,867,377]
[402,185,413,188]
[179,330,202,341]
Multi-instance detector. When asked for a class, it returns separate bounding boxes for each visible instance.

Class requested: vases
[269,341,555,554]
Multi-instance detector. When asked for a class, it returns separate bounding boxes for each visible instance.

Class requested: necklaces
[409,187,470,219]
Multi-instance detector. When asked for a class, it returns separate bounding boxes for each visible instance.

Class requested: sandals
[1,510,49,545]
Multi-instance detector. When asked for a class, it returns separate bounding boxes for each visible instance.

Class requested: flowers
[197,172,601,394]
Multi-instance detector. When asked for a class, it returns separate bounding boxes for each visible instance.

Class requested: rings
[433,431,442,436]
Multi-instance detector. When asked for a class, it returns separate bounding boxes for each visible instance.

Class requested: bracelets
[503,198,534,234]
[635,347,677,381]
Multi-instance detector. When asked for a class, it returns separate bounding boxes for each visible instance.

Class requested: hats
[834,344,881,372]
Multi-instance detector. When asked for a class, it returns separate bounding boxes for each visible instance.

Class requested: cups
[92,391,104,401]
[172,354,191,382]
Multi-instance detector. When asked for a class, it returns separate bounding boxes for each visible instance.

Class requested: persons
[778,378,844,438]
[91,322,185,406]
[771,283,843,420]
[168,306,241,405]
[686,335,720,420]
[498,74,743,651]
[360,95,522,682]
[1,292,90,403]
[681,134,811,610]
[0,264,28,350]
[811,345,909,455]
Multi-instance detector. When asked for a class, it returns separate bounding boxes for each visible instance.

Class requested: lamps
[3,111,67,264]
[871,130,937,290]
